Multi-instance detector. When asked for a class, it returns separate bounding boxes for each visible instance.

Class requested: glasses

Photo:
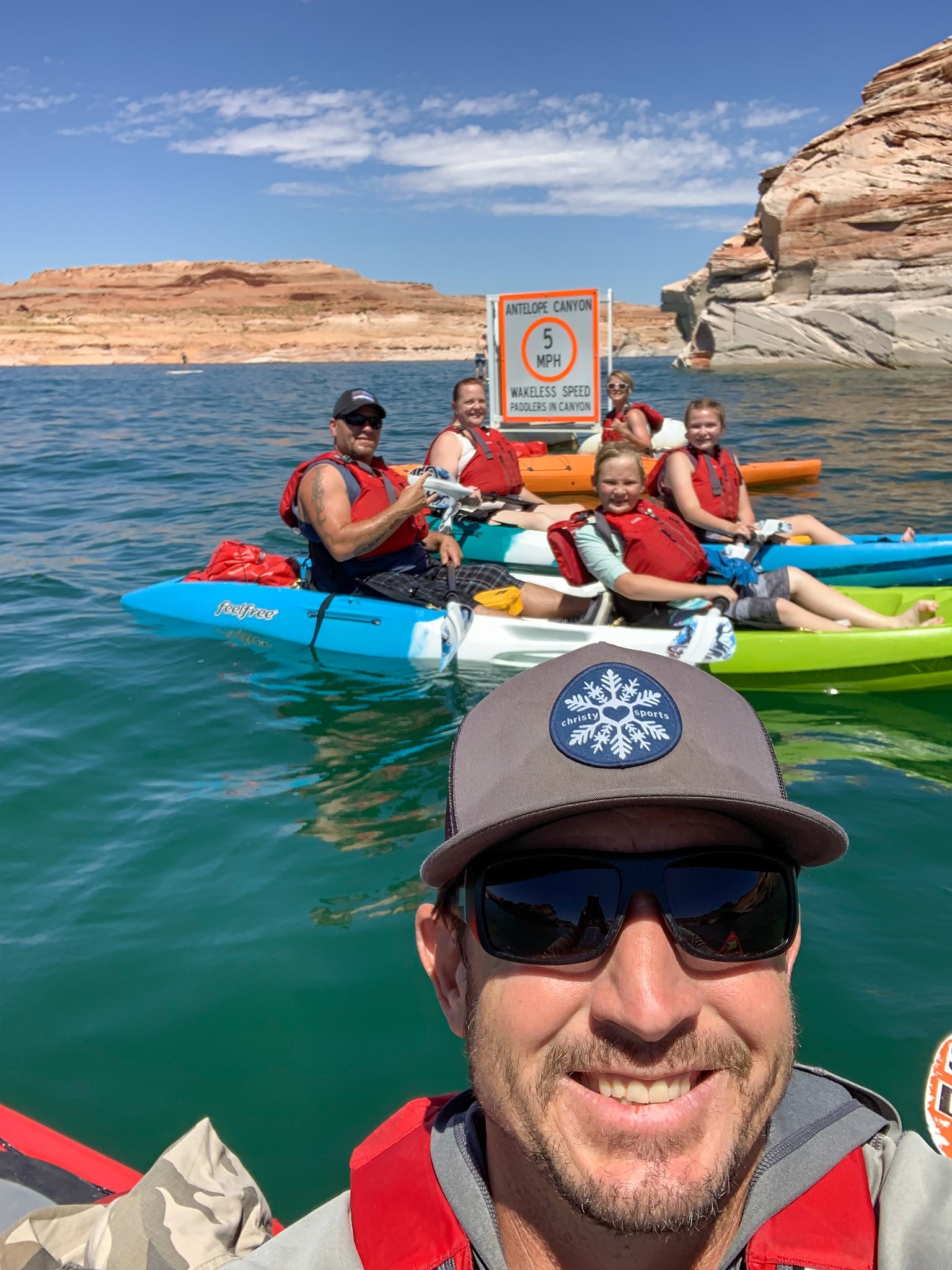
[606,382,629,391]
[336,412,383,430]
[446,838,800,965]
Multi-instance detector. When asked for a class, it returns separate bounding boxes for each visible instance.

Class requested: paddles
[437,502,474,674]
[666,519,794,667]
[407,465,535,508]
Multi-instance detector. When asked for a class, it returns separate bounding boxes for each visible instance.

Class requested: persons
[297,389,590,619]
[657,398,915,545]
[601,371,655,457]
[181,352,189,371]
[429,378,588,531]
[572,440,944,634]
[213,641,952,1270]
[474,334,488,378]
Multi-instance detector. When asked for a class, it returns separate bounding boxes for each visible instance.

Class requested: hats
[332,388,386,420]
[419,642,849,888]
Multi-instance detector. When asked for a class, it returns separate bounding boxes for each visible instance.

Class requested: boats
[427,510,951,589]
[678,348,714,369]
[119,553,951,701]
[388,452,822,496]
